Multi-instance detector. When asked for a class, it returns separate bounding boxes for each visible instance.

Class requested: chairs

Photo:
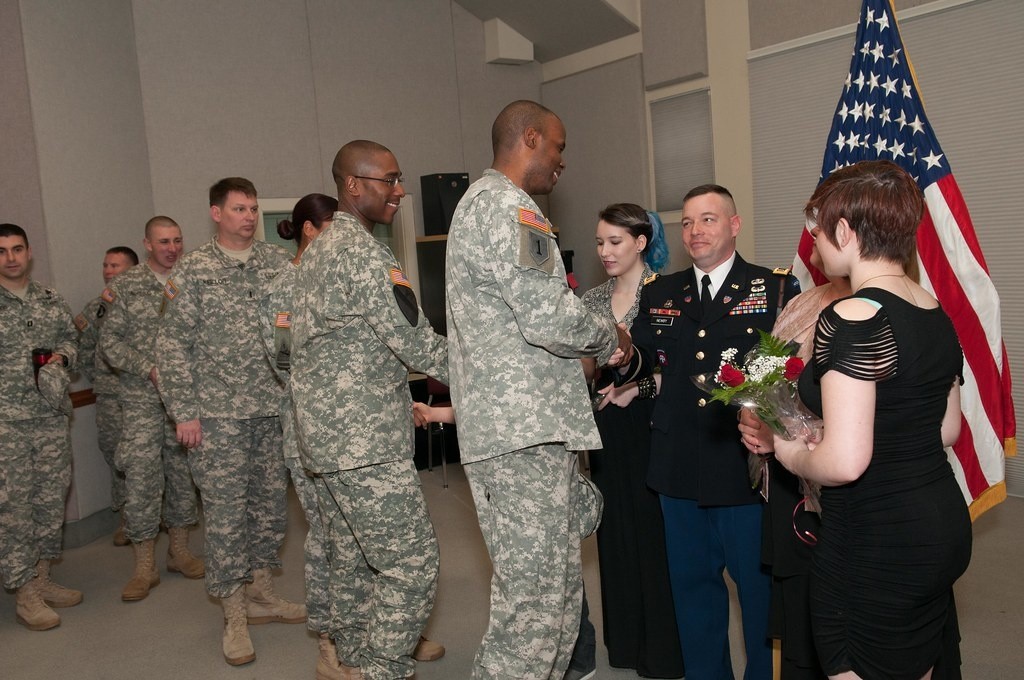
[427,372,454,489]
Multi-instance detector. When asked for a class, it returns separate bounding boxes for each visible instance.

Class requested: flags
[782,0,1019,523]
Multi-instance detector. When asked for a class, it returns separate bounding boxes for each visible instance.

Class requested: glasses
[354,175,404,188]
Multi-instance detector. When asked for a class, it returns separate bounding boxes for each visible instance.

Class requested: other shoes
[564,666,596,680]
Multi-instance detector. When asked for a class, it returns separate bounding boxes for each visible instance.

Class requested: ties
[700,275,713,319]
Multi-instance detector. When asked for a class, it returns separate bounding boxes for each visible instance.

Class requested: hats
[38,363,73,416]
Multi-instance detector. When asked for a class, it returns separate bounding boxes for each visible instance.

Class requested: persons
[412,202,670,680]
[276,139,448,680]
[154,176,310,666]
[447,97,633,680]
[0,223,85,630]
[608,183,802,680]
[736,159,970,680]
[77,215,204,603]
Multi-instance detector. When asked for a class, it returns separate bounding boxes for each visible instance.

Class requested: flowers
[707,328,823,545]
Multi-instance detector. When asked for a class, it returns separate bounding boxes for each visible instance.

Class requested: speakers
[421,173,470,235]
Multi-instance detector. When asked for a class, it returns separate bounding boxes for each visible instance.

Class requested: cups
[32,348,51,391]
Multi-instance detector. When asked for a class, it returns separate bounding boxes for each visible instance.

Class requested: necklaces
[855,272,909,292]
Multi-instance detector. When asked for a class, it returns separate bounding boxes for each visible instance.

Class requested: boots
[114,511,130,545]
[167,527,205,578]
[414,635,445,660]
[316,632,360,680]
[218,587,255,665]
[245,569,306,624]
[14,581,60,631]
[121,539,160,601]
[31,559,82,607]
